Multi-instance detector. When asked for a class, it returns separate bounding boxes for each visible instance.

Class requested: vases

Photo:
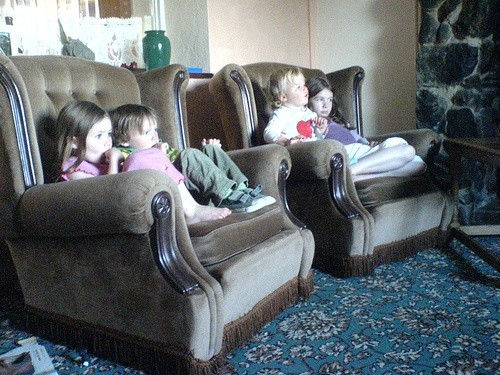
[144,29,171,71]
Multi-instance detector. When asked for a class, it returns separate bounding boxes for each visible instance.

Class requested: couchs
[208,62,456,280]
[0,47,317,375]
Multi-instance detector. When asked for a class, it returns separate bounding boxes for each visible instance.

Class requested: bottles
[0,16,20,56]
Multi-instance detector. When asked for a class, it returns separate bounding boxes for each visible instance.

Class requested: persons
[264,66,328,146]
[305,75,427,182]
[108,104,277,214]
[50,100,232,225]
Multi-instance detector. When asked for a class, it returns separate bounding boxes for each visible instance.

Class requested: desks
[443,137,500,278]
[189,71,214,151]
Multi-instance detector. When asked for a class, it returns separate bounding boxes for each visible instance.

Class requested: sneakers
[243,184,276,205]
[219,191,268,213]
